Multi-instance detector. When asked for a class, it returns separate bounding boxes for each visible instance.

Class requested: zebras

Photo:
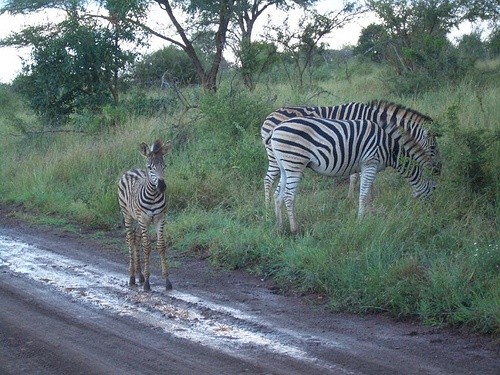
[264,116,437,234]
[118,138,173,292]
[260,99,441,210]
